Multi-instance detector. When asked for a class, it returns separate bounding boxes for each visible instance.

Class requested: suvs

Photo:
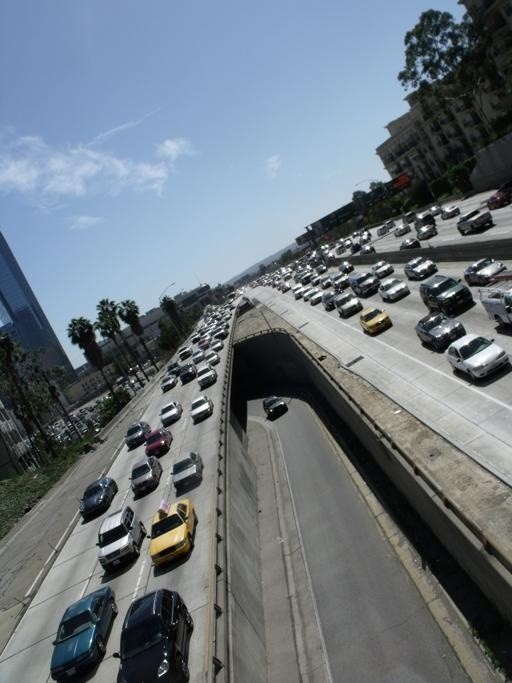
[96,507,146,573]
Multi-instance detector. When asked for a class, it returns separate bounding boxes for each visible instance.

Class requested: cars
[191,399,214,422]
[126,421,150,450]
[144,429,172,457]
[80,478,117,522]
[50,585,118,682]
[41,367,144,438]
[128,456,162,497]
[160,307,232,392]
[147,499,198,568]
[250,194,512,382]
[159,402,182,427]
[113,588,194,682]
[169,453,203,491]
[263,396,288,418]
